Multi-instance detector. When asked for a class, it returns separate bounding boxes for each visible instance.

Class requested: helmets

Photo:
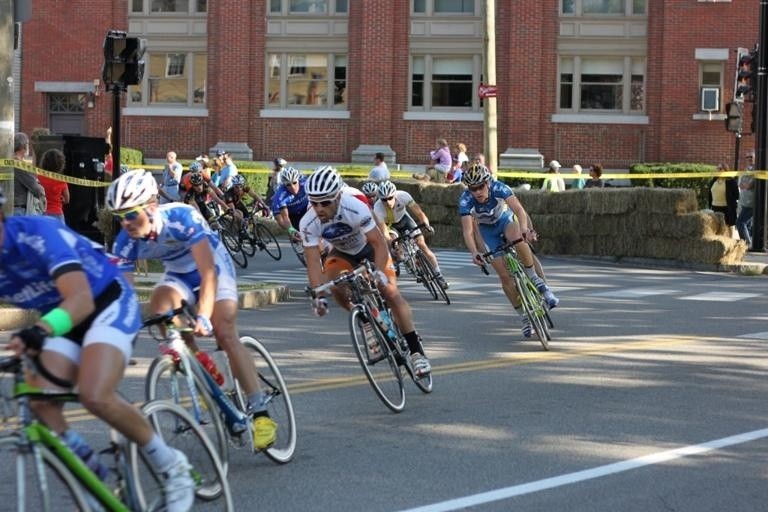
[461,163,493,187]
[280,167,301,185]
[104,169,157,213]
[189,161,202,172]
[231,175,245,185]
[189,173,203,186]
[304,165,344,200]
[274,158,287,167]
[377,181,398,200]
[362,182,379,197]
[216,148,226,156]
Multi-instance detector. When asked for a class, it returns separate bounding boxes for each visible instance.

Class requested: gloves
[208,204,271,230]
[10,323,47,354]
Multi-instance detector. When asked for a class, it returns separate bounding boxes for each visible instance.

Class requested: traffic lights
[108,35,138,86]
[738,56,753,99]
[139,38,148,87]
[727,103,740,133]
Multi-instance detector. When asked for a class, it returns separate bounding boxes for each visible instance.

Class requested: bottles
[379,307,397,340]
[60,430,110,482]
[193,350,226,386]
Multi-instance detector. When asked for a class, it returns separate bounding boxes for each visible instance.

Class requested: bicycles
[0,336,233,511]
[123,300,296,500]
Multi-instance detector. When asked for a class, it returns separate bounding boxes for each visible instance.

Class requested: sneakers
[252,413,277,452]
[436,273,450,290]
[361,320,385,364]
[88,469,126,511]
[158,447,196,511]
[192,393,208,420]
[410,349,432,377]
[529,273,559,310]
[521,317,533,338]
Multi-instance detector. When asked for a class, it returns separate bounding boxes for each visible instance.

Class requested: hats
[550,159,562,168]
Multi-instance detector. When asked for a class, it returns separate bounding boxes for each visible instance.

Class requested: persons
[734,151,755,247]
[102,165,279,454]
[708,161,739,238]
[298,168,433,383]
[0,187,197,512]
[457,162,557,338]
[11,129,606,247]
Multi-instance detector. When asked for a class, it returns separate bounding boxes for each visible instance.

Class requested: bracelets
[40,307,73,338]
